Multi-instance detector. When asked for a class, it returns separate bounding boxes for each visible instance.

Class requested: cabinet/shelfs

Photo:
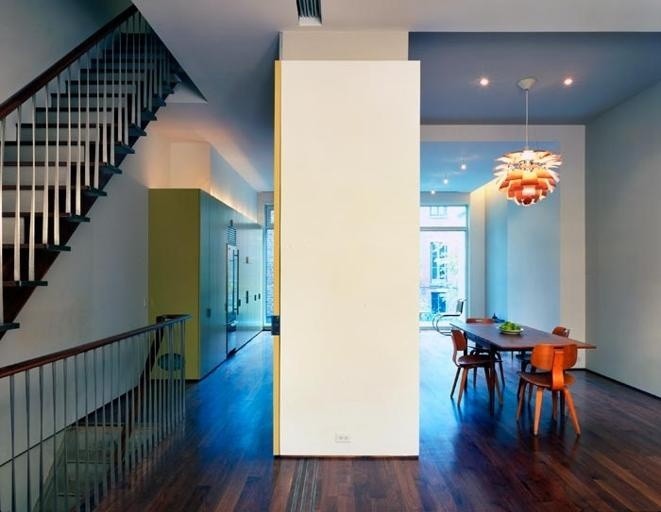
[147,187,265,382]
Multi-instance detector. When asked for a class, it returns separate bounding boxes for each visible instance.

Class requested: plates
[495,327,525,335]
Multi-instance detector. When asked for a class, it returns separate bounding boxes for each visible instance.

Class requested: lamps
[492,78,561,207]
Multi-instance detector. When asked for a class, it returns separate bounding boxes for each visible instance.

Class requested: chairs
[515,343,581,435]
[450,329,504,406]
[464,318,505,388]
[432,300,465,336]
[516,325,570,403]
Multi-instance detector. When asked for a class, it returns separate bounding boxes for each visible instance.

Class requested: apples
[500,322,520,330]
